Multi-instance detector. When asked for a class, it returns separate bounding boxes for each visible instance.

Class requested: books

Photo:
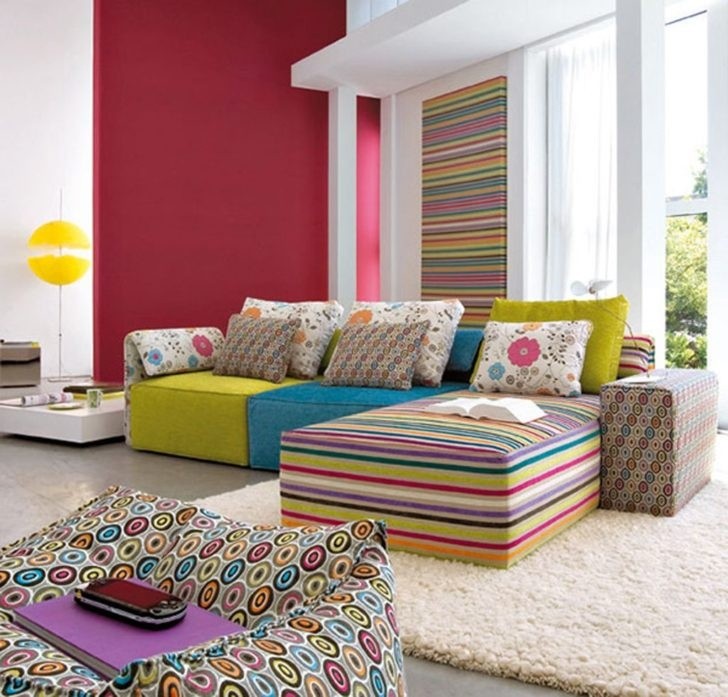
[424,398,548,425]
[13,579,249,680]
[0,392,74,406]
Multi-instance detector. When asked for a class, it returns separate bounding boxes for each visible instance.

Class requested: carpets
[183,427,728,697]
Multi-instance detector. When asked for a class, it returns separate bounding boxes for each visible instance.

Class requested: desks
[0,395,126,442]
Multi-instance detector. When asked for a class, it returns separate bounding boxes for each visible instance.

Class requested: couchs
[279,336,656,570]
[123,315,485,472]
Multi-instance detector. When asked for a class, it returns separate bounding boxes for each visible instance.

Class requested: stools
[0,484,406,697]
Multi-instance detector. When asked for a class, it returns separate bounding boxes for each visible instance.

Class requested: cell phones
[73,578,188,630]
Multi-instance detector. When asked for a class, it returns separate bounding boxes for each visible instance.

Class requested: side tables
[600,369,719,517]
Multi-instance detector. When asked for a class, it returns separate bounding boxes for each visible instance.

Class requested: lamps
[591,277,666,384]
[30,191,93,381]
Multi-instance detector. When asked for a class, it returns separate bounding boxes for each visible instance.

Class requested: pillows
[216,297,629,397]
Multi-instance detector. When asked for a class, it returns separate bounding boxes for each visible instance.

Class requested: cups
[86,388,103,408]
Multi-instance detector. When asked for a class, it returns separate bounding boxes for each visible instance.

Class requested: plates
[48,402,83,410]
